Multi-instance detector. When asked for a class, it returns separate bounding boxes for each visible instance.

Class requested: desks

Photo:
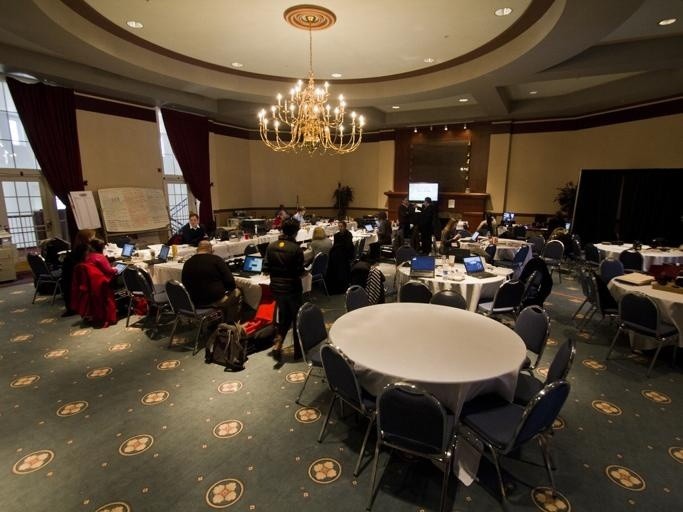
[325,301,528,450]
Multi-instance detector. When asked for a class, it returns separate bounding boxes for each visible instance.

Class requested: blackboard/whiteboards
[97,186,170,232]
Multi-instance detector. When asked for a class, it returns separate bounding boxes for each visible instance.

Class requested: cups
[492,236,498,245]
[243,234,248,240]
[171,244,177,258]
[448,255,455,266]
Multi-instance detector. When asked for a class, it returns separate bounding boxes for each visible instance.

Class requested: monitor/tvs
[408,181,439,203]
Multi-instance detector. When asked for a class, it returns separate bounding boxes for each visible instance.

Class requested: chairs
[315,342,378,478]
[549,231,683,379]
[287,298,331,405]
[400,216,548,314]
[16,200,399,376]
[453,374,570,510]
[366,378,463,512]
[510,336,578,473]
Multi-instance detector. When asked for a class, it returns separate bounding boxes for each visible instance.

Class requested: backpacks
[206,321,247,371]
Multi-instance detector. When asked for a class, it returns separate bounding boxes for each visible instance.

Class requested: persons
[69,228,141,315]
[270,209,290,229]
[369,212,392,260]
[416,197,436,255]
[440,213,496,263]
[182,240,243,324]
[398,197,415,238]
[332,221,355,257]
[308,227,332,264]
[263,219,305,361]
[290,208,309,227]
[180,213,209,245]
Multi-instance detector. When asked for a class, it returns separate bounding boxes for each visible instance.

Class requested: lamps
[251,3,366,158]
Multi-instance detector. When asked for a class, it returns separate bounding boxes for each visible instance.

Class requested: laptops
[503,212,514,224]
[240,256,264,276]
[365,224,375,233]
[408,257,435,278]
[144,245,170,266]
[463,256,496,279]
[460,231,479,242]
[114,243,135,261]
[449,247,470,263]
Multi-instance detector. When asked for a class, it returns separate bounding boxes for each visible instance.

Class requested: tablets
[112,262,128,275]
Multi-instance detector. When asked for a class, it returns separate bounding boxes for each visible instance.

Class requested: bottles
[441,255,446,265]
[441,263,448,281]
[148,249,155,260]
